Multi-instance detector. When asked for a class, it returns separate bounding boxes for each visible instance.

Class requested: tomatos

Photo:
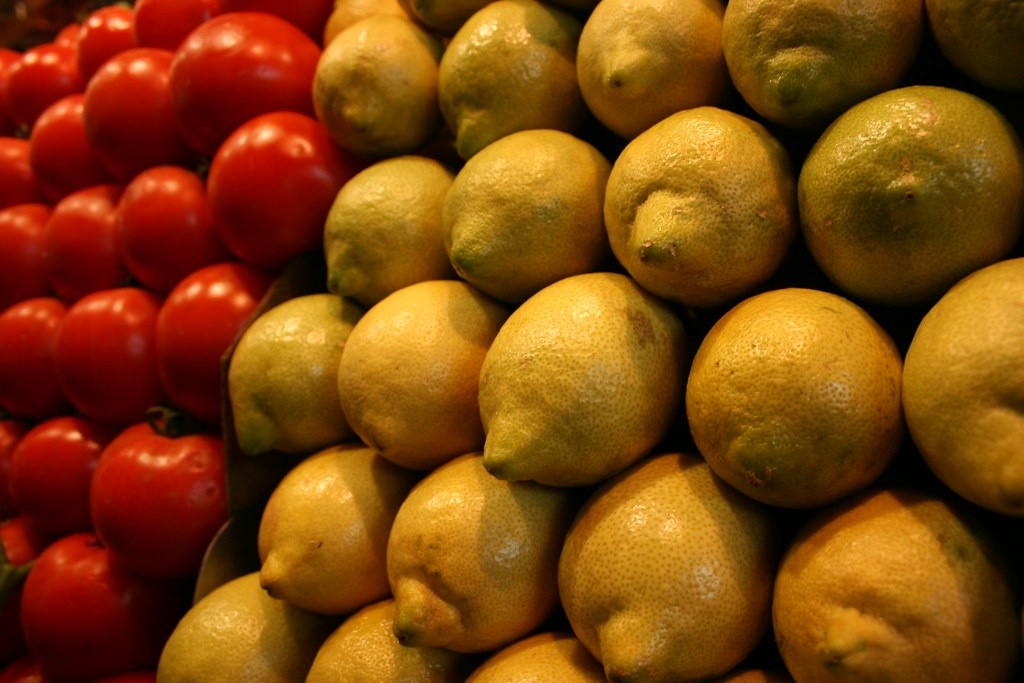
[0,0,345,683]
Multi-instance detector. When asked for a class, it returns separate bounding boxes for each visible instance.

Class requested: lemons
[155,0,1024,683]
